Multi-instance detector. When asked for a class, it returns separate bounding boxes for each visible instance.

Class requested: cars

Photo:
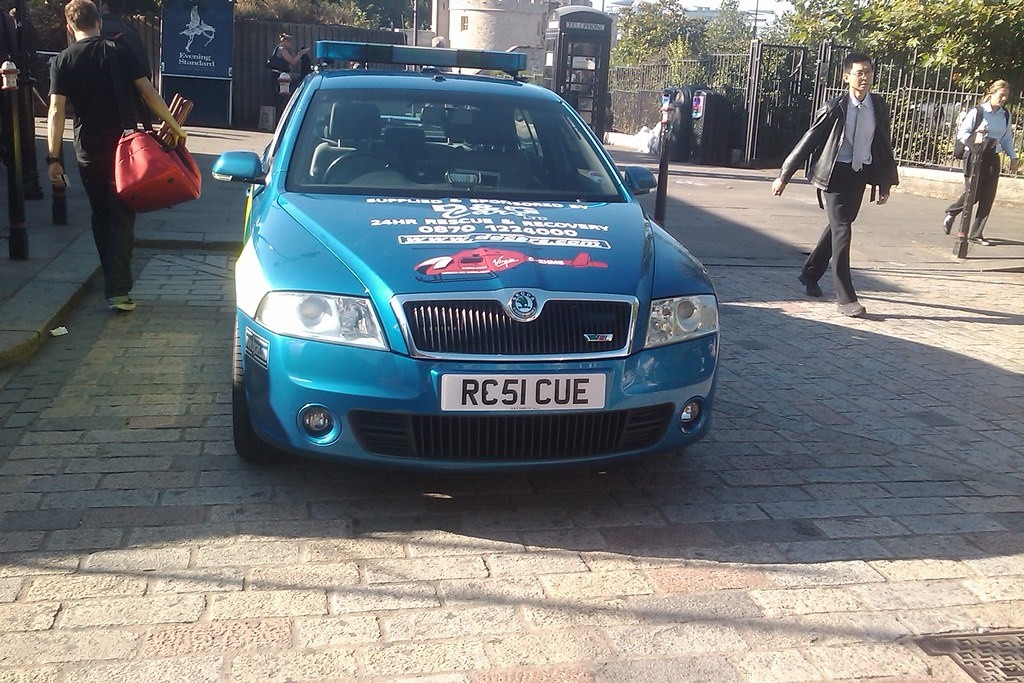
[211,39,722,485]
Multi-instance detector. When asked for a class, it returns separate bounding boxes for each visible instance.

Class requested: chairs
[464,112,539,191]
[442,108,478,144]
[384,129,430,184]
[307,103,391,185]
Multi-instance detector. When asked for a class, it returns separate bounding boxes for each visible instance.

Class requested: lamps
[975,129,990,145]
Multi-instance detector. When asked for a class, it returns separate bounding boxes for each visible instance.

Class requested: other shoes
[969,236,991,246]
[107,295,136,311]
[798,272,822,297]
[943,213,956,235]
[836,301,866,317]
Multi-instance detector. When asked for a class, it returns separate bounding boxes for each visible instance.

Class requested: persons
[422,35,453,72]
[943,80,1020,248]
[771,50,901,320]
[98,0,152,129]
[934,101,944,130]
[46,0,187,311]
[0,6,44,200]
[268,31,308,127]
[300,47,311,82]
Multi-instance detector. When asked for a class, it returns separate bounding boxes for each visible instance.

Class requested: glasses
[849,70,874,77]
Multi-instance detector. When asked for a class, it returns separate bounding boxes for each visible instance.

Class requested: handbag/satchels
[114,125,202,213]
[267,45,292,73]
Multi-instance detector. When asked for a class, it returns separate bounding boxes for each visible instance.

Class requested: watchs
[46,155,61,164]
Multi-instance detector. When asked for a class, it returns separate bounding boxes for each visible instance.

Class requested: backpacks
[953,105,1010,160]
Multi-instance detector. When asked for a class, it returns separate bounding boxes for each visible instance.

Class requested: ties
[851,104,865,173]
